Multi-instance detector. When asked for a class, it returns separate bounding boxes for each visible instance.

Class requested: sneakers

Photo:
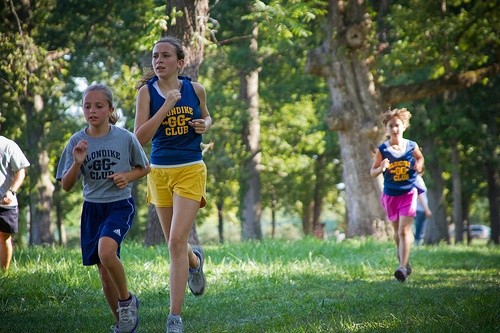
[167,315,182,333]
[397,255,412,276]
[394,266,407,281]
[109,292,139,333]
[188,246,205,296]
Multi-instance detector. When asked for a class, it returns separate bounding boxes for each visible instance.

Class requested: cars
[462,225,491,240]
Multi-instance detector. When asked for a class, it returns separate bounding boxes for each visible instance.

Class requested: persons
[134,38,212,333]
[415,167,431,244]
[0,135,30,269]
[316,223,345,244]
[371,108,424,282]
[56,85,151,333]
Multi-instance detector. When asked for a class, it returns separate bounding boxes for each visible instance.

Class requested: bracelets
[8,188,16,195]
